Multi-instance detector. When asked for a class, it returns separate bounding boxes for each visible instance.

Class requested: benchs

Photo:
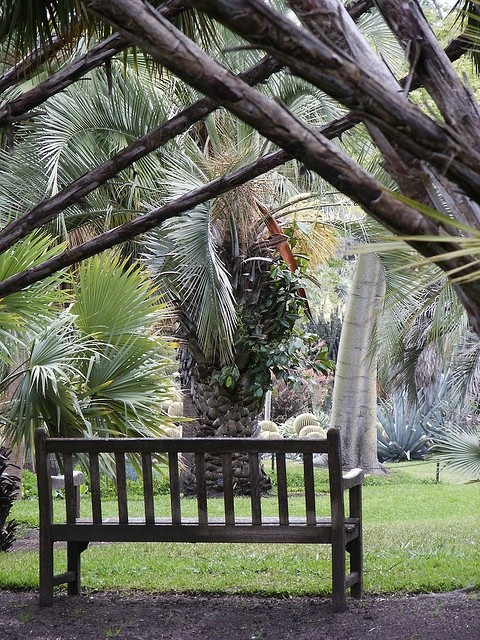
[34,428,365,612]
